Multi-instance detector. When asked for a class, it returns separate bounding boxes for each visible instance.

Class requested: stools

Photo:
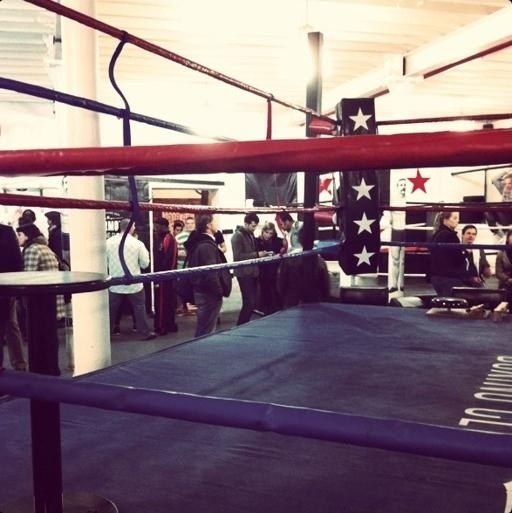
[0,272,118,513]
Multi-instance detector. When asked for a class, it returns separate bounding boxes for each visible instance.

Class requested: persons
[0,209,68,373]
[498,231,512,323]
[492,169,512,201]
[429,211,482,295]
[106,210,327,340]
[460,223,492,284]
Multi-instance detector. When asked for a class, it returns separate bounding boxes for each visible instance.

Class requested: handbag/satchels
[173,275,196,305]
[59,259,70,271]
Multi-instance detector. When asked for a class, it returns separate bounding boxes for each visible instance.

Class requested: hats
[17,208,60,240]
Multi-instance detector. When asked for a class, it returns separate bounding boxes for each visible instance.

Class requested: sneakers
[111,325,157,341]
[57,316,73,327]
